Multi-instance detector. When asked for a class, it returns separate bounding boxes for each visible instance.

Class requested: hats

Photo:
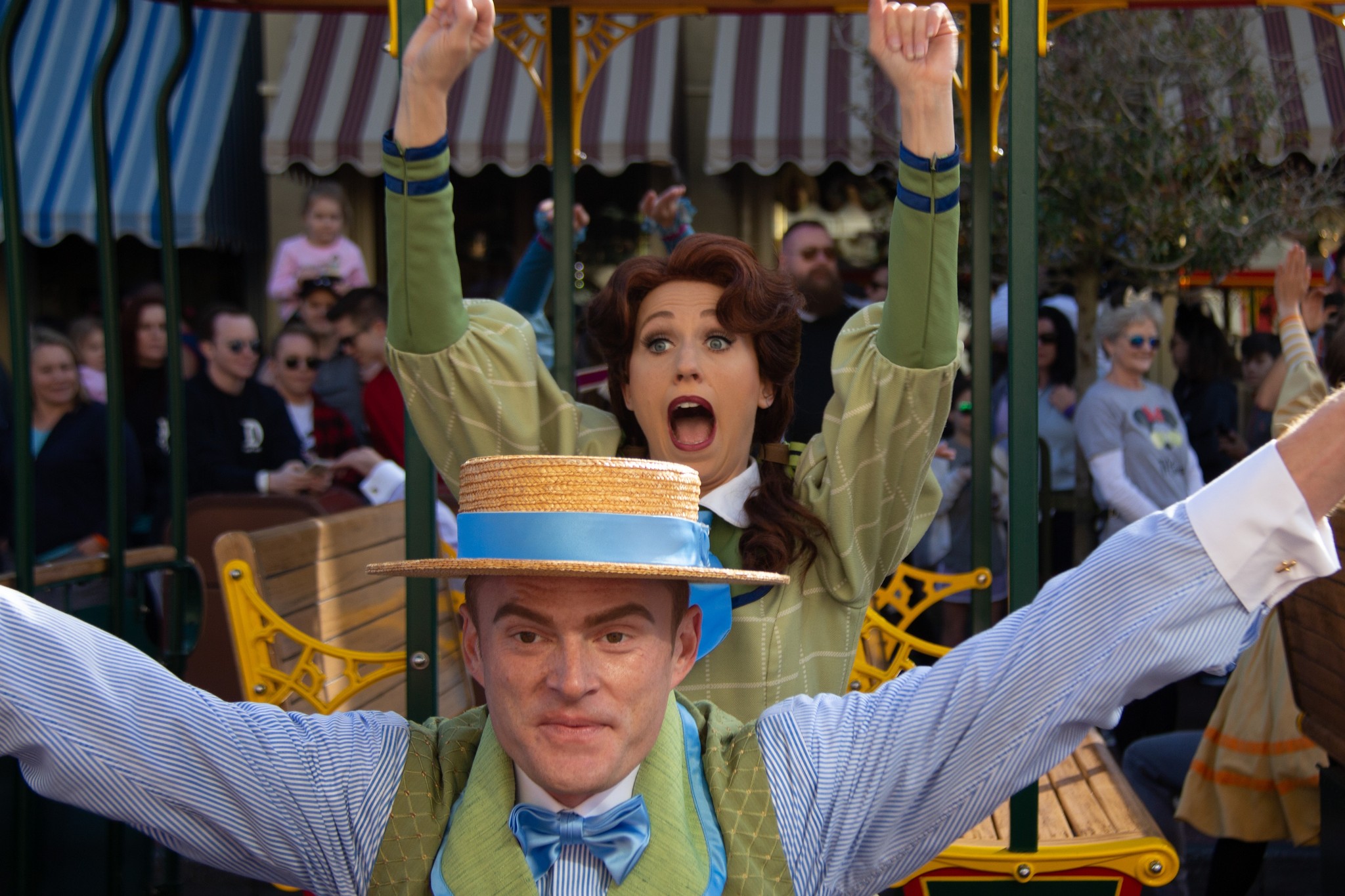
[1040,295,1079,333]
[364,454,789,587]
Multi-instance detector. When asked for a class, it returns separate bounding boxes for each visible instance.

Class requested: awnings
[259,11,906,179]
[1059,4,1345,167]
[0,0,265,249]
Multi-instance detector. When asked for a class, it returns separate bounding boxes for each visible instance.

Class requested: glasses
[337,324,369,354]
[787,246,840,264]
[210,339,261,355]
[273,355,323,371]
[1117,333,1162,349]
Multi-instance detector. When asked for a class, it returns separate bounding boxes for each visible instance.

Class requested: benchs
[212,502,1178,896]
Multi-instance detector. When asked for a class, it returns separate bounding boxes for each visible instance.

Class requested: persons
[381,0,958,724]
[0,384,1345,896]
[0,180,465,634]
[501,182,892,476]
[909,243,1344,896]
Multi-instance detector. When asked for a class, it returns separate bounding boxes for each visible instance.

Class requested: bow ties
[688,509,734,661]
[507,795,652,886]
